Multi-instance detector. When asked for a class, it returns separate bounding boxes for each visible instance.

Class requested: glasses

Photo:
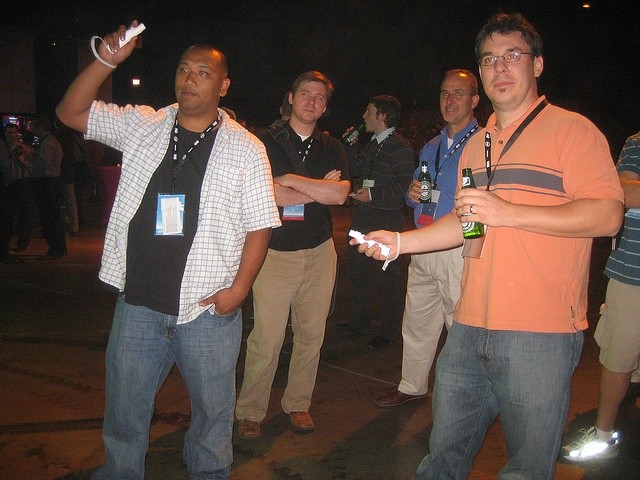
[479,51,533,66]
[438,90,475,100]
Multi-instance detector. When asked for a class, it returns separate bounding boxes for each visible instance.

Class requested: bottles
[417,161,432,204]
[341,121,365,147]
[460,169,483,238]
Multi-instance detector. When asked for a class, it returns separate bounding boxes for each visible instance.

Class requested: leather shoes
[288,411,315,431]
[334,322,363,335]
[62,251,67,256]
[37,254,64,260]
[375,391,428,408]
[236,420,262,439]
[12,246,26,252]
[366,335,395,349]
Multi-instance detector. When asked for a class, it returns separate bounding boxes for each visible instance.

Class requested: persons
[57,126,83,238]
[54,19,283,478]
[373,66,483,410]
[347,14,625,480]
[235,67,352,440]
[19,113,68,258]
[4,122,36,251]
[336,92,416,353]
[561,131,639,465]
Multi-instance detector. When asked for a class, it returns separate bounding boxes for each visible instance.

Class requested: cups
[352,178,363,193]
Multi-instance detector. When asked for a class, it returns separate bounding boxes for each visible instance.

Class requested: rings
[469,205,473,213]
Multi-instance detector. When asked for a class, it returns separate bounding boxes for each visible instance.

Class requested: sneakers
[558,426,620,462]
